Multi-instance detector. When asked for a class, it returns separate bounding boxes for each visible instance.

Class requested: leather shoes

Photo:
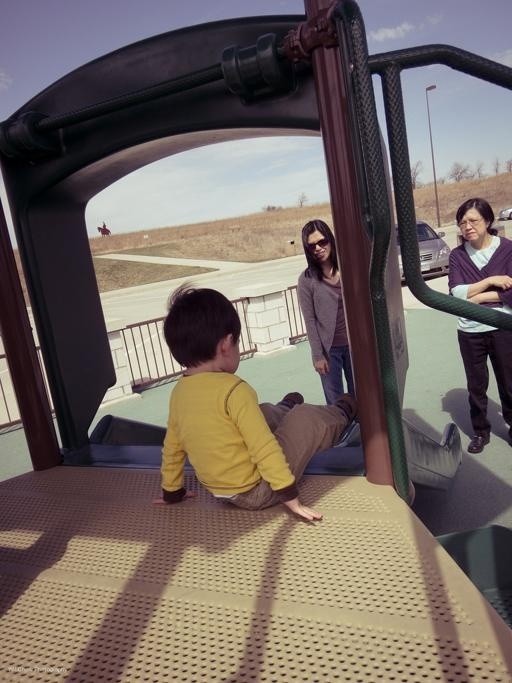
[467,432,490,453]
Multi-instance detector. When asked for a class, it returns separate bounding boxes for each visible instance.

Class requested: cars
[393,218,452,285]
[498,207,512,220]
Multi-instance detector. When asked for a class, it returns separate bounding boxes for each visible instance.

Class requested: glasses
[458,217,484,230]
[308,237,329,252]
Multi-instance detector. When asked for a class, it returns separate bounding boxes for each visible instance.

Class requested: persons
[447,197,512,453]
[295,218,355,404]
[103,221,106,228]
[149,279,357,523]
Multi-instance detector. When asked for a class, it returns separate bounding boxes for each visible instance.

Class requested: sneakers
[283,391,304,409]
[331,391,361,448]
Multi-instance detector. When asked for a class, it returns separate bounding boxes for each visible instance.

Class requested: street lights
[425,84,442,228]
